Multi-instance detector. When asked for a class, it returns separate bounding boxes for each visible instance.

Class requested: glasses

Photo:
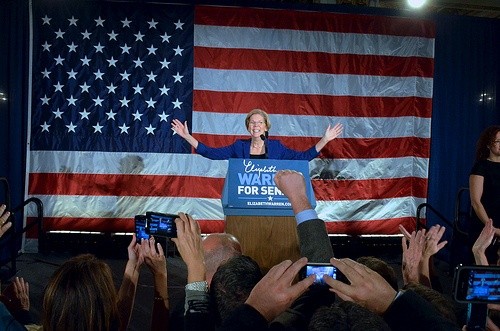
[248,121,264,126]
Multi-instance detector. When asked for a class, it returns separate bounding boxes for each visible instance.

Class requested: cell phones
[299,262,351,289]
[452,264,500,304]
[145,211,183,238]
[134,215,151,245]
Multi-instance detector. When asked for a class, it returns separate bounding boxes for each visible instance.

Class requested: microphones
[260,134,269,159]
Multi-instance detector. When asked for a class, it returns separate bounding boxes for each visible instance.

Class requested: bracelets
[152,297,170,302]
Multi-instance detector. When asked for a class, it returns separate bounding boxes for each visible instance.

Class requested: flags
[24,7,437,237]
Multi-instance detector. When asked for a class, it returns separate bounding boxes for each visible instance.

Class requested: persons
[466,128,500,268]
[171,109,344,163]
[0,168,500,331]
